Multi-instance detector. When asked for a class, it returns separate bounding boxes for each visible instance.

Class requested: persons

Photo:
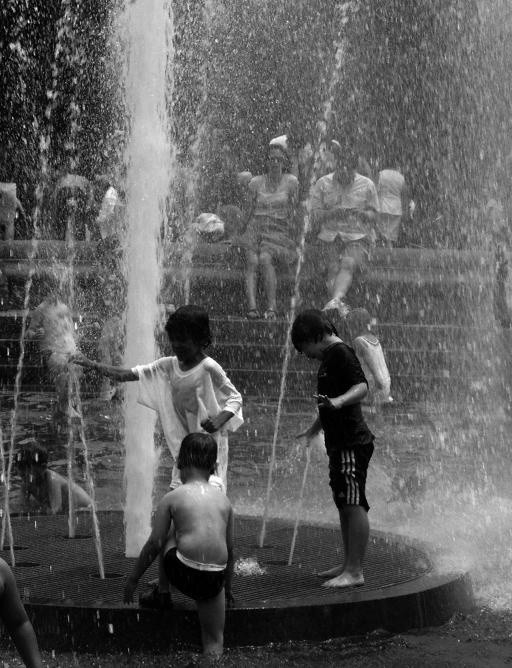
[67,304,246,585]
[345,310,394,403]
[196,137,407,247]
[122,430,237,655]
[87,175,123,249]
[221,144,301,320]
[289,308,376,588]
[13,441,93,515]
[54,157,95,236]
[310,149,380,317]
[26,270,78,411]
[0,558,43,668]
[96,235,128,400]
